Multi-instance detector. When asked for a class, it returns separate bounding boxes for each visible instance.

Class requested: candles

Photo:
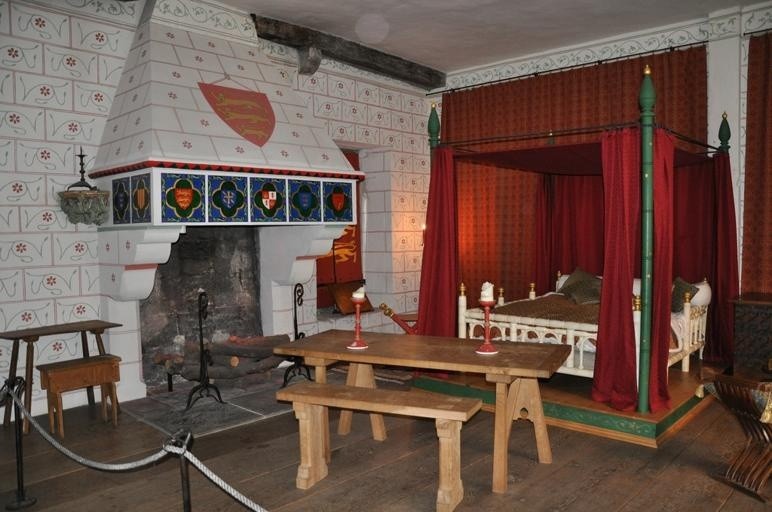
[351,285,366,299]
[480,280,495,302]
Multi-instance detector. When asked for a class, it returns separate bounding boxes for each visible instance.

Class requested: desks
[271,327,573,494]
[0,319,123,436]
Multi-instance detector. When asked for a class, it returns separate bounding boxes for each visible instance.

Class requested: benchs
[35,353,121,441]
[275,382,484,511]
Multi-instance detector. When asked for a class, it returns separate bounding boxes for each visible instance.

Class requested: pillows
[559,268,700,315]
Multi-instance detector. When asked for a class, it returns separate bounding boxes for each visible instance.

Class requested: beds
[454,268,711,395]
